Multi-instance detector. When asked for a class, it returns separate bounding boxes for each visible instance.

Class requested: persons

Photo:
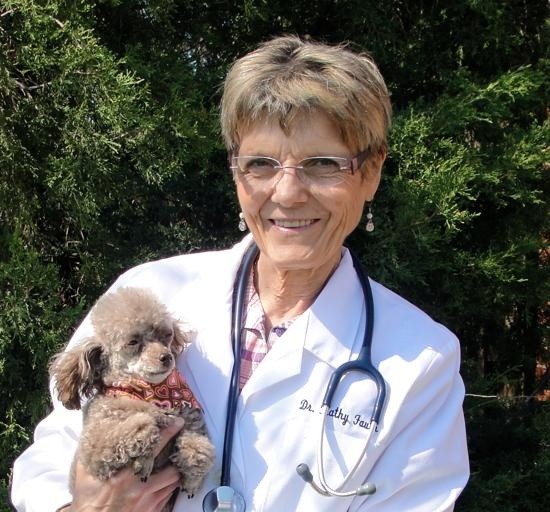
[9,34,472,512]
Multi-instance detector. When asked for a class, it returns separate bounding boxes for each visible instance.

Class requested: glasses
[224,146,373,182]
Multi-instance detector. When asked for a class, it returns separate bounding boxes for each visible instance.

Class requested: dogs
[45,287,220,512]
